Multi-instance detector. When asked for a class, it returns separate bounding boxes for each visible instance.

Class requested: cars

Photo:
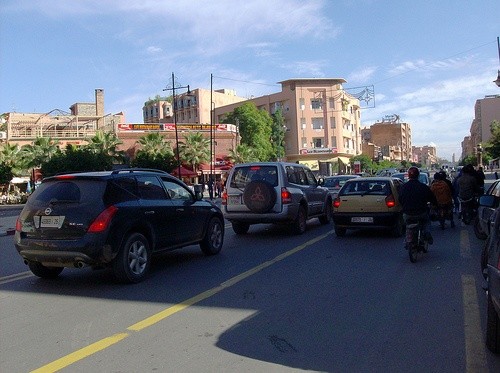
[332,176,406,239]
[320,175,361,200]
[390,172,431,188]
[477,171,500,359]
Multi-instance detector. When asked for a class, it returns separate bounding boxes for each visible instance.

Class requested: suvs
[220,161,333,237]
[14,168,225,284]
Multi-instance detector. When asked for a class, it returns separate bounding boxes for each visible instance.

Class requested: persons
[430,164,486,229]
[398,167,438,248]
[206,177,223,201]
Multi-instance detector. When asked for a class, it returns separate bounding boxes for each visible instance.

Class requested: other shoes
[425,231,433,246]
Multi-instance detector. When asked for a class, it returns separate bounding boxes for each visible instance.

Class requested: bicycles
[404,202,430,263]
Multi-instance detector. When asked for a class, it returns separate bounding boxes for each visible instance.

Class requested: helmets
[407,166,420,177]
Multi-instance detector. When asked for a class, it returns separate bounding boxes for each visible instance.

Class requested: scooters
[437,204,447,230]
